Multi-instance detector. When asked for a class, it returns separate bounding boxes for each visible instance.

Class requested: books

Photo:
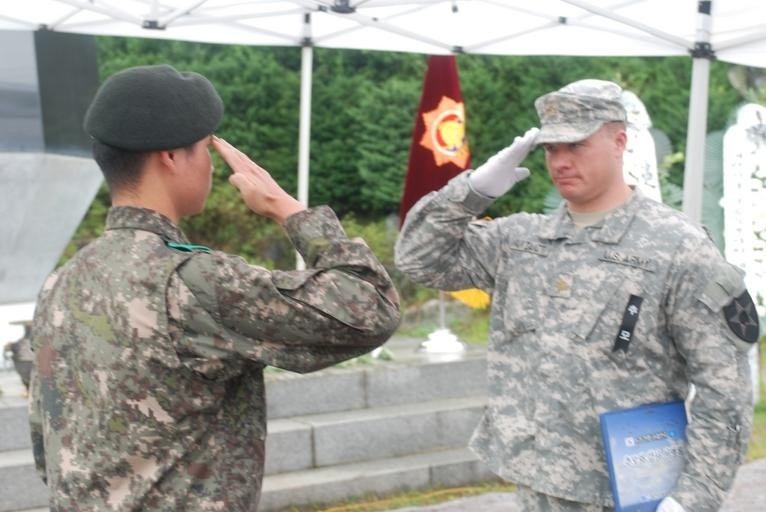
[598,396,689,512]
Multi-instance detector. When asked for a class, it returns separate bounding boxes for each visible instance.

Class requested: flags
[398,53,475,236]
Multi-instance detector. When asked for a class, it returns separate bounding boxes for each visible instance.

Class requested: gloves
[467,127,542,201]
[655,495,685,512]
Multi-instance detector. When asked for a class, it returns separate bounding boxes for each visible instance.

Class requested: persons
[392,77,761,512]
[22,64,404,512]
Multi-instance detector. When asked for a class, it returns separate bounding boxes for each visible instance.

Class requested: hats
[534,79,625,144]
[82,63,225,152]
